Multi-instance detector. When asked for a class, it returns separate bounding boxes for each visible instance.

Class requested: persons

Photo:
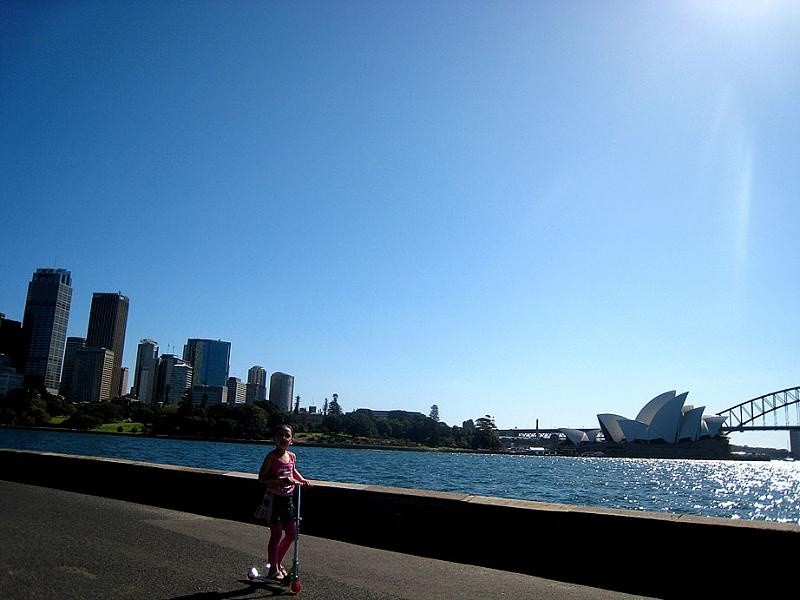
[258,423,314,581]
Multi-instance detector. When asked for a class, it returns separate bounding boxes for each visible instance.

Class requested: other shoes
[279,567,288,576]
[268,571,284,580]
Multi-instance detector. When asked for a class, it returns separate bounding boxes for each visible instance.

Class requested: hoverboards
[246,481,307,596]
